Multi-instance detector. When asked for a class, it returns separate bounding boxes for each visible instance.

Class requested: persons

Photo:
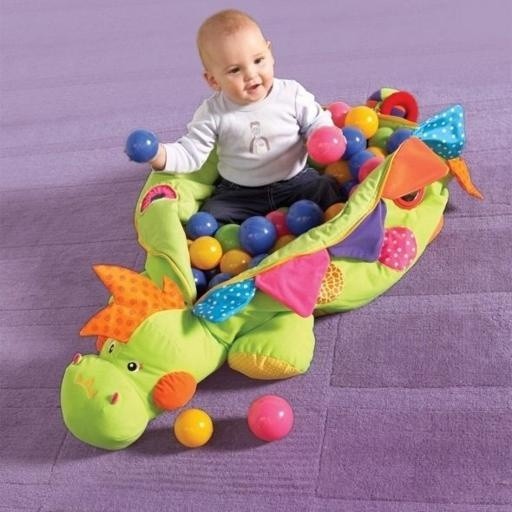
[124,9,349,282]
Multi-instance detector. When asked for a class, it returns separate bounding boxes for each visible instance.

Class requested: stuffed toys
[61,86,484,451]
